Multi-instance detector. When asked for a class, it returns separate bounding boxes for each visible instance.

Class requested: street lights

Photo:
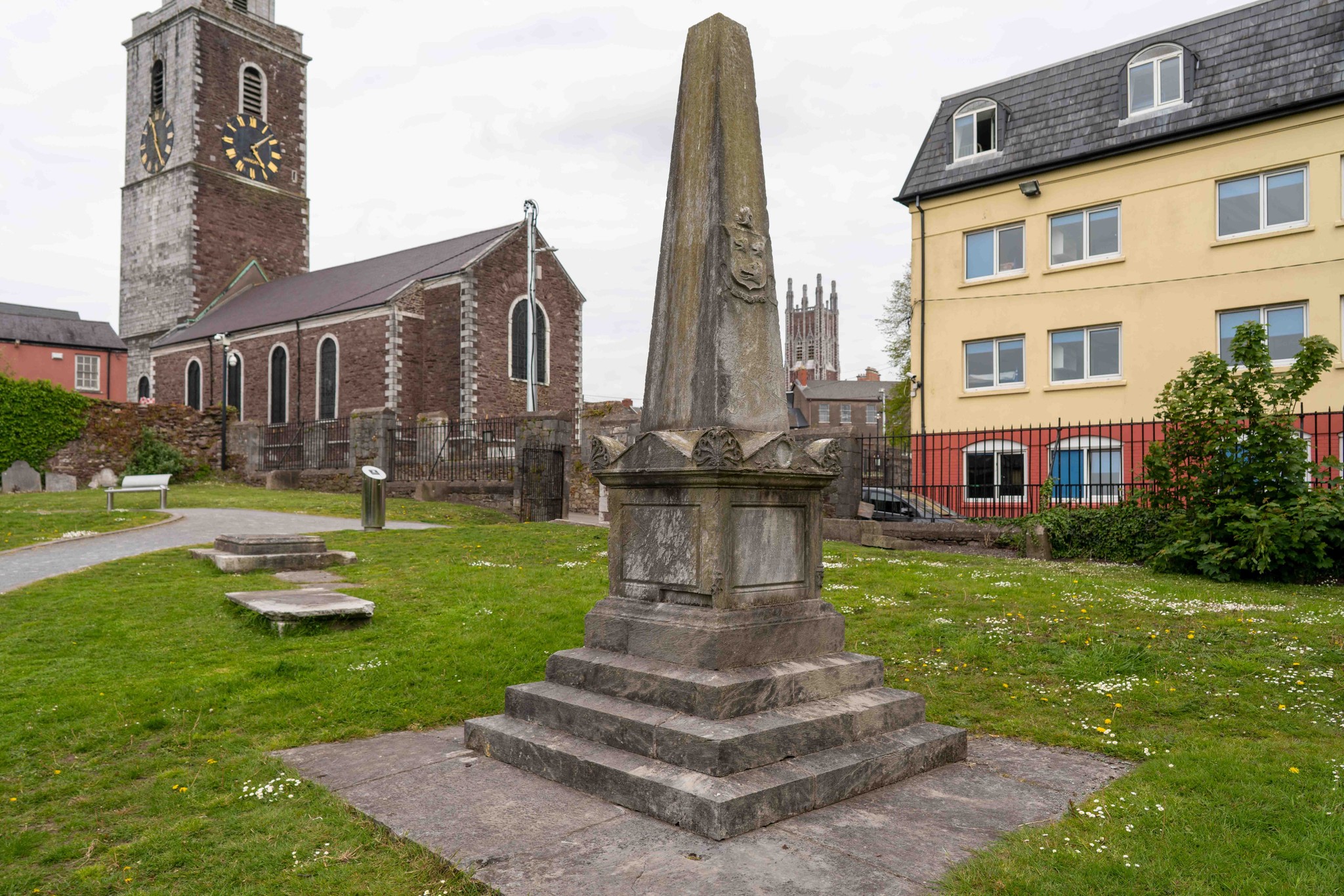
[877,387,886,449]
[214,330,237,470]
[522,199,559,409]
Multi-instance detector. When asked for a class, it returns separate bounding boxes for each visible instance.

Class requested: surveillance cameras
[214,335,221,341]
[906,372,917,378]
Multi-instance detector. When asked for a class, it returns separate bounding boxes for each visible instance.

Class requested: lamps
[1020,180,1042,196]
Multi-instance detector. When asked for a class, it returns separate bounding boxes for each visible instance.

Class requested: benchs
[105,474,172,510]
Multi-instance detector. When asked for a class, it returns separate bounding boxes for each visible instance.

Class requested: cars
[855,486,969,522]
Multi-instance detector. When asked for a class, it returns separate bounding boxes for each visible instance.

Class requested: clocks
[224,112,283,183]
[141,108,176,173]
